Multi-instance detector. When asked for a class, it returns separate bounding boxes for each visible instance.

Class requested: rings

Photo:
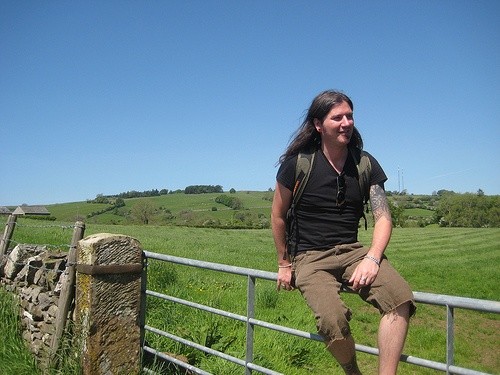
[361,277,367,281]
[282,284,285,289]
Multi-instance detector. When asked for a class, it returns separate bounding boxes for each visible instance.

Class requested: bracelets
[279,264,292,268]
[365,255,380,265]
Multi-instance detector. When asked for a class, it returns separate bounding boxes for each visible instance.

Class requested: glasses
[336,173,346,206]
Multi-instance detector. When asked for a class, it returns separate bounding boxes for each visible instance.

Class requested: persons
[271,91,418,375]
